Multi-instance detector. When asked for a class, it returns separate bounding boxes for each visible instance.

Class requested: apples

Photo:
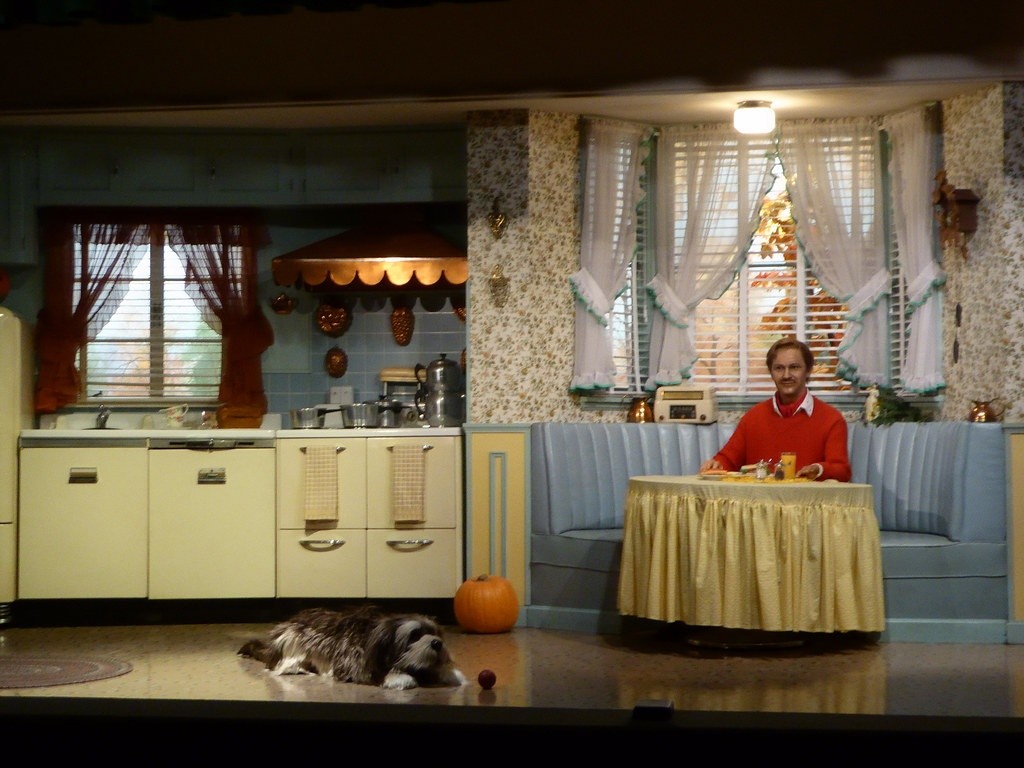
[479,670,497,688]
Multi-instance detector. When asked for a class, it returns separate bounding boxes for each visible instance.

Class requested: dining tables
[619,476,884,649]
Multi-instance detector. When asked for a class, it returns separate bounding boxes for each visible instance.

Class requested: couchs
[532,421,1008,643]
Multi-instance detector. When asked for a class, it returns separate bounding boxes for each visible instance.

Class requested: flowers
[864,382,934,429]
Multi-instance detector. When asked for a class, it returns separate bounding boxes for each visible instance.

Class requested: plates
[698,475,726,481]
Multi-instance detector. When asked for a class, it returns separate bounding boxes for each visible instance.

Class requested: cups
[160,403,189,416]
[163,416,187,429]
[781,451,797,480]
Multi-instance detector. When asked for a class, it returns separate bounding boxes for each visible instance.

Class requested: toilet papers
[260,413,282,429]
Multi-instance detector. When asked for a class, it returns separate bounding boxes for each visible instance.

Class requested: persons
[700,337,852,482]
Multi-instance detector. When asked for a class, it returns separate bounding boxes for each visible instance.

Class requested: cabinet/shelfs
[19,448,276,598]
[276,437,462,596]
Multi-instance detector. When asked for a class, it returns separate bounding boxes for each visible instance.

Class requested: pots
[289,407,342,429]
[339,403,402,429]
[364,395,402,428]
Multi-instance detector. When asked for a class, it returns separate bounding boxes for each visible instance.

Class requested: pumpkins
[453,574,519,634]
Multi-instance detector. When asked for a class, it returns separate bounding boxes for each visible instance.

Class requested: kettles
[414,390,466,428]
[414,354,466,393]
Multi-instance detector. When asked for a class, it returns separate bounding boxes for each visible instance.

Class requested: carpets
[0,654,134,687]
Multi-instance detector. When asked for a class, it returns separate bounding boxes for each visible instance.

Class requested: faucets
[81,404,121,430]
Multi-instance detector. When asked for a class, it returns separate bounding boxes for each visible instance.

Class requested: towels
[392,444,427,524]
[303,444,338,522]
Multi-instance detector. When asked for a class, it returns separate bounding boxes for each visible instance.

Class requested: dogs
[239,603,469,691]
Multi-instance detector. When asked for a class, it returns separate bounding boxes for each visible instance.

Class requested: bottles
[756,461,768,480]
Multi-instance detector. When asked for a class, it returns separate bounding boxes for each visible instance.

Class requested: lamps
[733,100,776,133]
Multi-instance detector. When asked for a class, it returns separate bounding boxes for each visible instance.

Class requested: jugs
[620,395,653,423]
[969,397,1005,423]
